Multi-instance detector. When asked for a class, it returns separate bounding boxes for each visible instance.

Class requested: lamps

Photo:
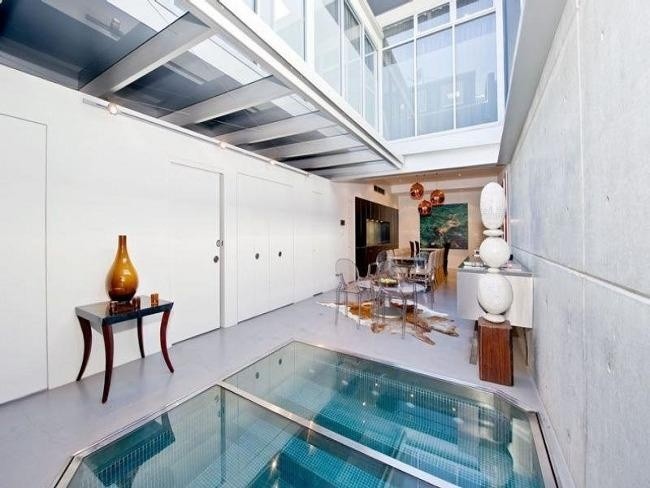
[410,173,444,217]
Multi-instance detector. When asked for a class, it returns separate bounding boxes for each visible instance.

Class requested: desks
[74,295,175,404]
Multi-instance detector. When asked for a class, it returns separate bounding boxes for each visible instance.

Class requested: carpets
[319,297,459,345]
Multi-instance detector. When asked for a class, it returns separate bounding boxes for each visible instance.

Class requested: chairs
[334,247,446,340]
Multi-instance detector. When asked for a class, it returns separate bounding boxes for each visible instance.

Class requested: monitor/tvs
[366,218,390,247]
